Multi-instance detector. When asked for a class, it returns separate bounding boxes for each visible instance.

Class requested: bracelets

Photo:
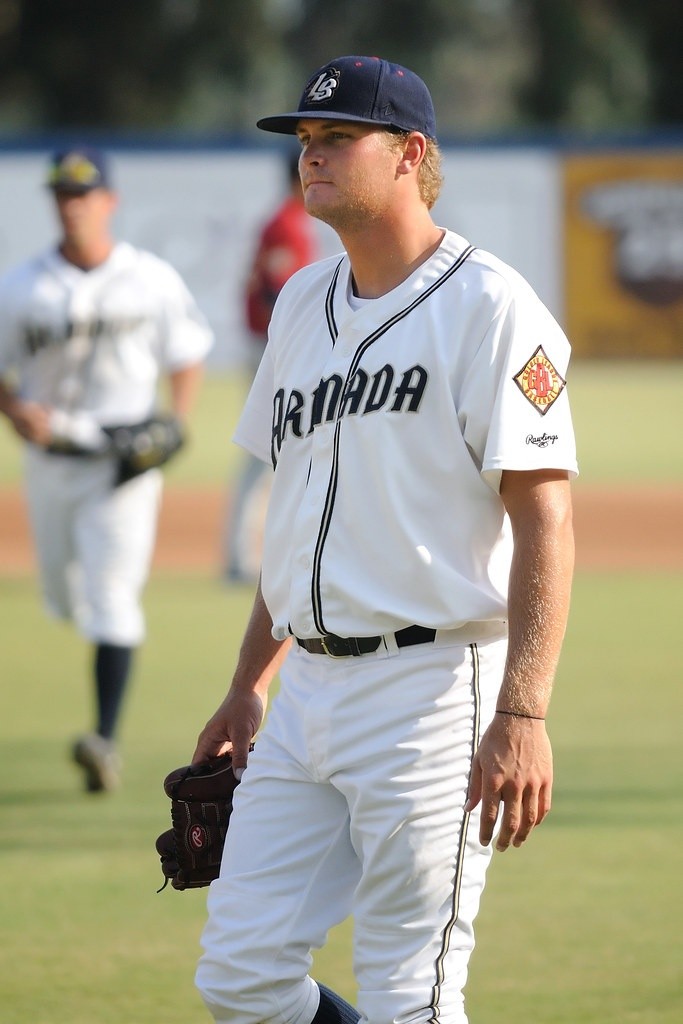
[495,710,545,720]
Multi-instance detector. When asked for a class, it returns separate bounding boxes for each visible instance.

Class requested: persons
[0,144,216,792]
[191,58,579,1024]
[223,144,322,581]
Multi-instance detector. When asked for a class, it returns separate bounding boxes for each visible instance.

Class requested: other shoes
[75,735,122,792]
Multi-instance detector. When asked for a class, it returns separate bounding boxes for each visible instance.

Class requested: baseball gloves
[102,415,181,487]
[156,746,251,893]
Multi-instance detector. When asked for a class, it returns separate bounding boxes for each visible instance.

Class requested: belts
[295,625,436,659]
[43,444,95,458]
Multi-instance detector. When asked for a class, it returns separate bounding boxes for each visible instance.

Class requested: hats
[255,55,438,141]
[42,140,109,190]
